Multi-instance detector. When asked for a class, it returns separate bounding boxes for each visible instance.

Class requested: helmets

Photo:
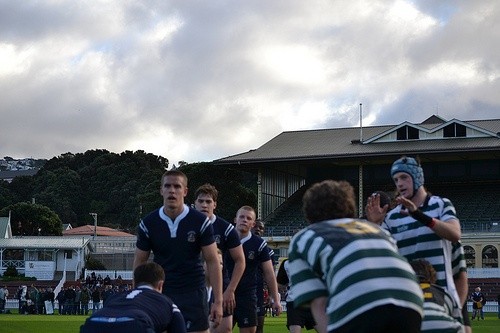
[390,157,424,193]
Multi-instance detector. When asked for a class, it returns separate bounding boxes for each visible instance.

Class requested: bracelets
[412,209,435,229]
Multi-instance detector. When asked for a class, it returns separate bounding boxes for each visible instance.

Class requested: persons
[410,258,466,333]
[233,205,283,333]
[79,263,188,333]
[17,282,118,315]
[252,219,275,333]
[480,287,486,320]
[192,183,246,333]
[288,180,424,333]
[0,285,8,314]
[365,156,462,325]
[471,287,483,321]
[81,272,110,287]
[131,170,223,333]
